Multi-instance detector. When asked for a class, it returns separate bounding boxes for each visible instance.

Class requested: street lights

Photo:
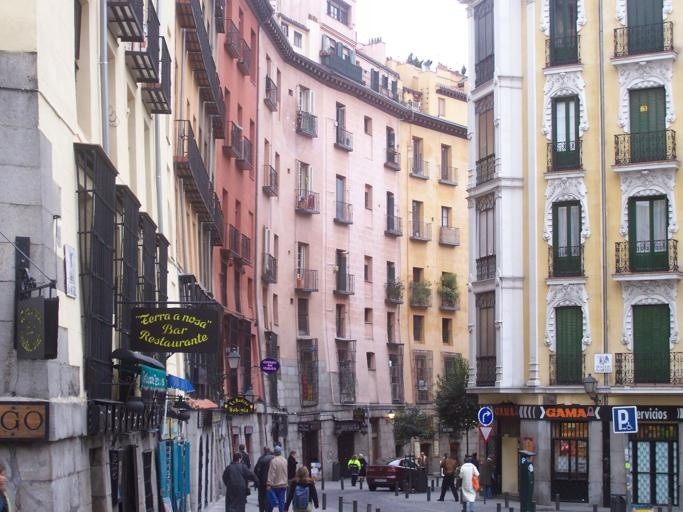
[264,487,279,512]
[472,464,481,491]
[350,464,359,475]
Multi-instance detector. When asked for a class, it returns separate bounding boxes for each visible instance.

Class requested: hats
[364,455,429,493]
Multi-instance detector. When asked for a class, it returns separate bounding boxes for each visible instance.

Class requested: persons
[418,452,427,466]
[357,453,367,482]
[348,454,360,486]
[436,452,500,512]
[220,442,320,511]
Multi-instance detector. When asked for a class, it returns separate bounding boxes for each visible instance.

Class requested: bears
[519,450,536,512]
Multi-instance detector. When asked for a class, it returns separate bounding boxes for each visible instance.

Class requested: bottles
[273,445,282,455]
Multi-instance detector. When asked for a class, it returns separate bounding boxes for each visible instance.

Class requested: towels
[611,495,625,512]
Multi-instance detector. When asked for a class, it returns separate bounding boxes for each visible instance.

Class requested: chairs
[437,499,444,501]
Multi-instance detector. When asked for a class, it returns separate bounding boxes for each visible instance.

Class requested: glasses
[385,410,395,425]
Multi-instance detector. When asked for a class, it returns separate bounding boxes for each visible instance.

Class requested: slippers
[292,482,310,511]
